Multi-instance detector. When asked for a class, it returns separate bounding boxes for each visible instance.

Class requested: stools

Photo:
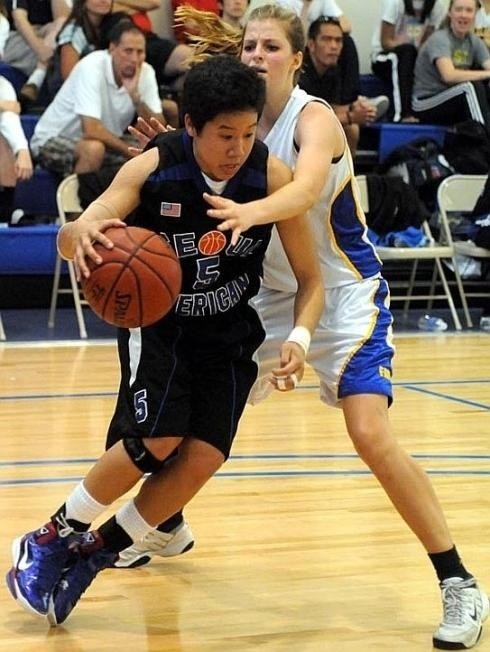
[374,115,453,170]
[0,217,71,276]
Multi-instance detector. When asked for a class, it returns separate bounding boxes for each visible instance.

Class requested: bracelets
[285,325,311,355]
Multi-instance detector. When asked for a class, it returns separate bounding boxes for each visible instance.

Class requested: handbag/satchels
[380,136,456,213]
[443,120,490,175]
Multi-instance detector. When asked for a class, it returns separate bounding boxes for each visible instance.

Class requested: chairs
[45,177,93,339]
[429,172,489,328]
[348,176,461,331]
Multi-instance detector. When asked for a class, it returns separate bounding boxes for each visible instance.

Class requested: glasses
[318,16,340,24]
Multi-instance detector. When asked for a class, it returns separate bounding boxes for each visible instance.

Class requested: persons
[108,4,489,651]
[2,54,326,627]
[30,23,165,204]
[2,1,489,229]
[406,0,490,127]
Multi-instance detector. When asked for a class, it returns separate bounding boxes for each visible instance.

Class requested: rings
[277,379,287,390]
[289,373,298,388]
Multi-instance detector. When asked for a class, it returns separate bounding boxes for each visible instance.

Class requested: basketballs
[80,227,182,329]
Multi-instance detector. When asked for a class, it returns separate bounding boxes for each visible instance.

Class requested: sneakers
[20,80,41,101]
[47,531,120,628]
[6,522,84,617]
[356,94,390,125]
[433,576,489,650]
[114,519,194,567]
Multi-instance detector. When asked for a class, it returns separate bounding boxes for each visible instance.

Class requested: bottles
[418,314,448,331]
